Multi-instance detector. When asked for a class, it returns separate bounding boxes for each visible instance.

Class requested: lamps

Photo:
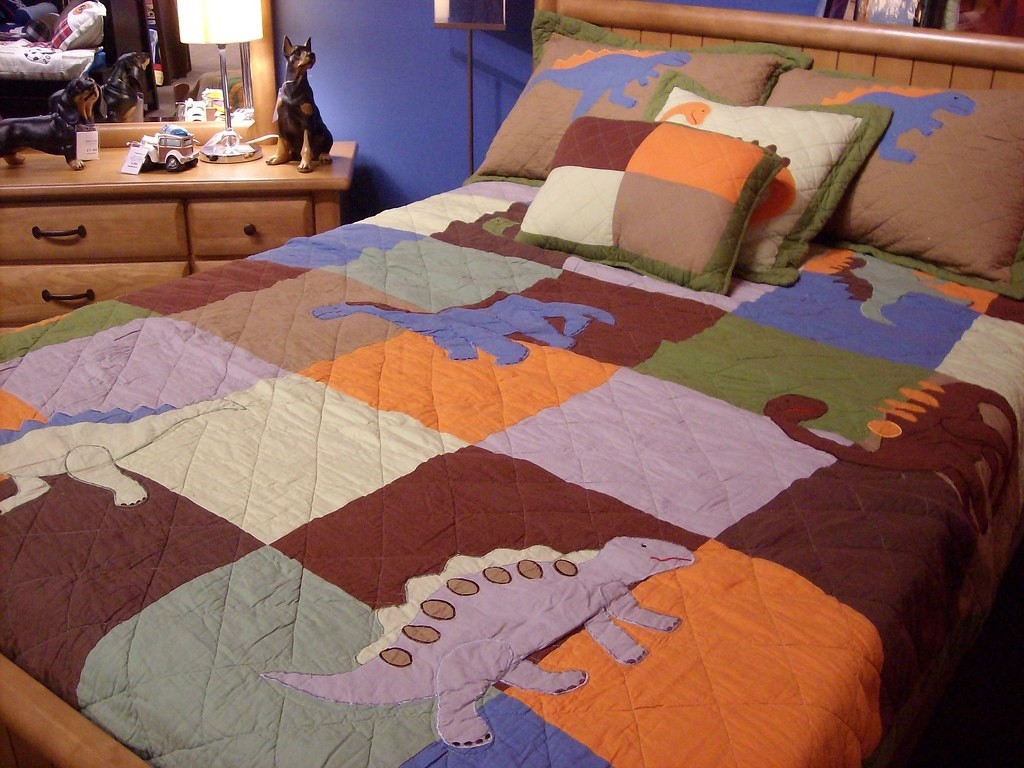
[177,0,263,167]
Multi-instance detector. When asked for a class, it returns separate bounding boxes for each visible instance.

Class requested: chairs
[146,87,175,122]
[174,79,189,102]
[187,78,202,100]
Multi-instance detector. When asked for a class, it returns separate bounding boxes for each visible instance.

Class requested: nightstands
[0,141,358,335]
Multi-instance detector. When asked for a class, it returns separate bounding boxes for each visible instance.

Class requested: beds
[0,0,159,124]
[0,1,1024,768]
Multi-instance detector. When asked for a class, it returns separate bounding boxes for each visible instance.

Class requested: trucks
[126,126,200,172]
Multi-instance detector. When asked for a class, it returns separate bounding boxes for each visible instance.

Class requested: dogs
[266,36,333,172]
[0,76,100,170]
[92,52,150,122]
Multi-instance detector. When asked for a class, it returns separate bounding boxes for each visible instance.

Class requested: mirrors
[88,0,254,124]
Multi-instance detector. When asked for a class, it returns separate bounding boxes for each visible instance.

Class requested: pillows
[50,0,106,51]
[462,9,1024,296]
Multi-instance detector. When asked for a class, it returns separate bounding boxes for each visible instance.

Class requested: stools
[185,98,207,122]
